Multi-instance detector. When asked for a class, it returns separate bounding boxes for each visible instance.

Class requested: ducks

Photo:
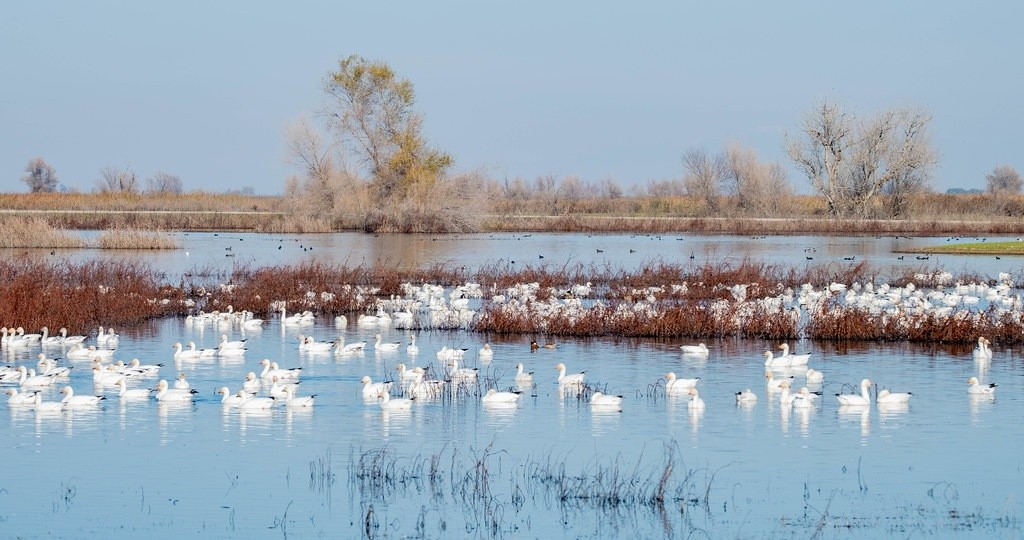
[0,272,1024,412]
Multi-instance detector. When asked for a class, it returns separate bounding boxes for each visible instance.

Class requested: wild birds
[23,234,1024,264]
